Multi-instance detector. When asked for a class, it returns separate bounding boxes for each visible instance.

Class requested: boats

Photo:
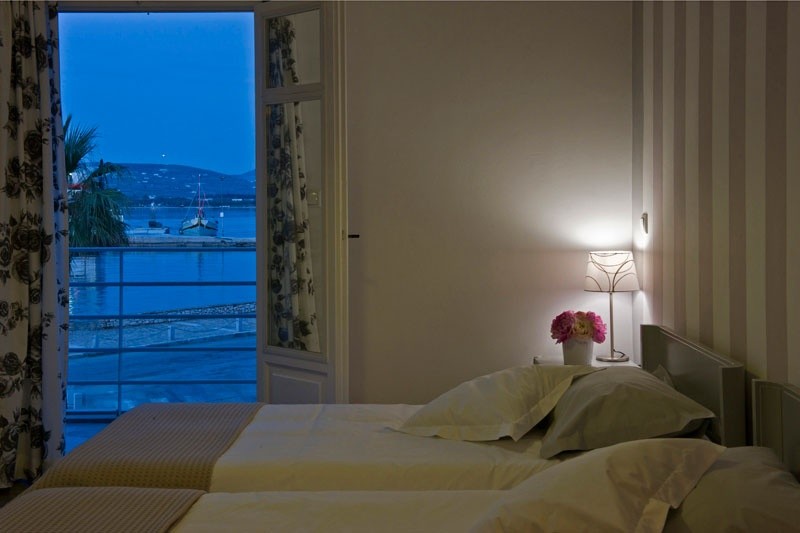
[148,221,163,228]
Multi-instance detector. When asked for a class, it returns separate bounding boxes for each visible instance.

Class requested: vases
[563,335,594,365]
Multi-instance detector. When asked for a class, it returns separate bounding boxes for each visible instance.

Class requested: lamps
[584,251,640,362]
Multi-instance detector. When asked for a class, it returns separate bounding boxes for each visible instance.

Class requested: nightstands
[534,356,641,368]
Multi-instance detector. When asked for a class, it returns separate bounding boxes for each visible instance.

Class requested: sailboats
[178,174,219,236]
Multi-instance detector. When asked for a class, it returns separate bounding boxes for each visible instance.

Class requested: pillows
[469,438,726,533]
[539,364,716,459]
[662,447,800,533]
[384,363,607,442]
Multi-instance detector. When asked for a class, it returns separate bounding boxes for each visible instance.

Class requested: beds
[0,325,800,533]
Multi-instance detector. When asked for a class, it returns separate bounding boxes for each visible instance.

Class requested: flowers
[549,309,607,344]
